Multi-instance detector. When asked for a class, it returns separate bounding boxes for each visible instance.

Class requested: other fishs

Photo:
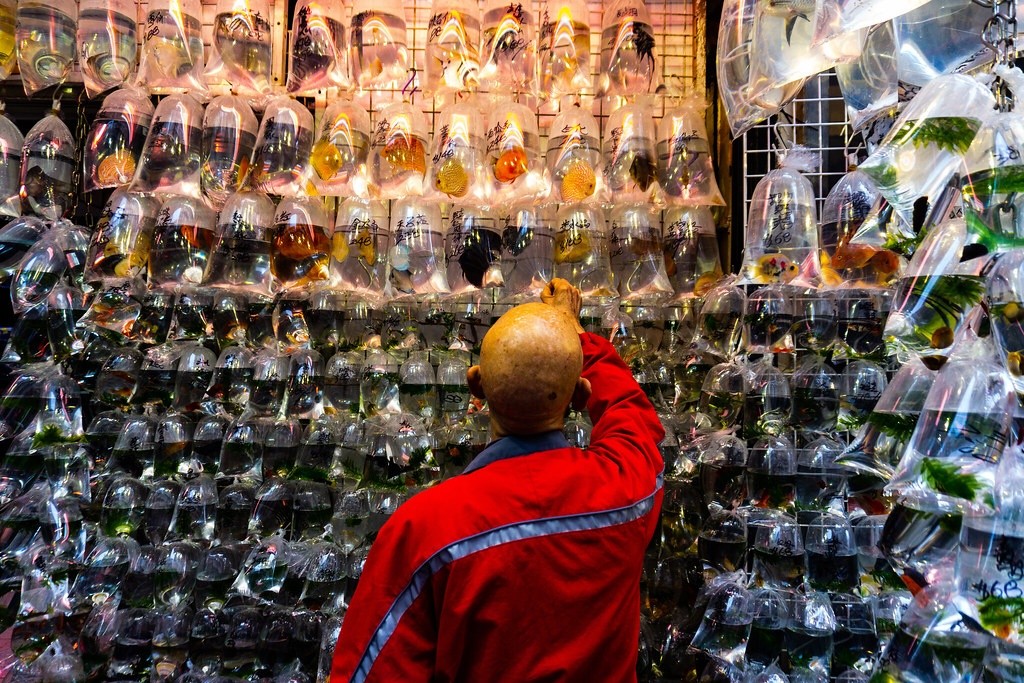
[0,0,1024,683]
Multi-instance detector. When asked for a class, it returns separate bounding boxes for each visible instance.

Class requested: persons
[325,275,664,683]
